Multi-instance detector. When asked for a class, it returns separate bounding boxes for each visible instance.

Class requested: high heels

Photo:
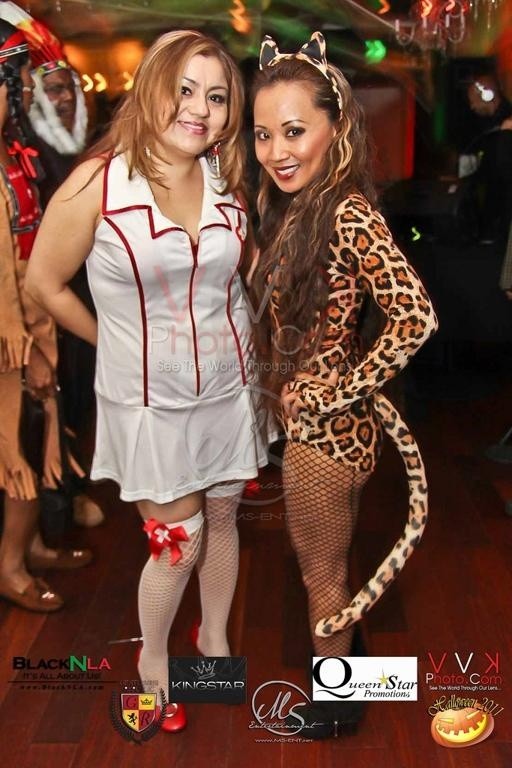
[283,697,366,741]
[132,645,189,732]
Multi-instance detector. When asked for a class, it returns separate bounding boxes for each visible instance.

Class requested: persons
[0,17,440,740]
[468,78,512,301]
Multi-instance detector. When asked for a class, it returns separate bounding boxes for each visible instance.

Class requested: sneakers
[0,577,65,614]
[25,543,94,572]
